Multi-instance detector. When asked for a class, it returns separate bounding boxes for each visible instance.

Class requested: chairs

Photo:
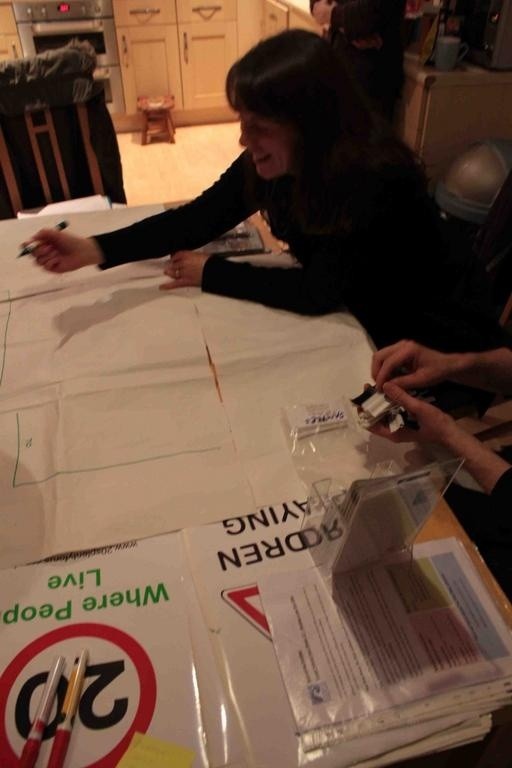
[2,42,105,219]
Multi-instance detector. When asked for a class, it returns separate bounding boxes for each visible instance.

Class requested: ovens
[11,1,127,115]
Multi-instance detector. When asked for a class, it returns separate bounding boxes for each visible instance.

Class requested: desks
[0,205,511,767]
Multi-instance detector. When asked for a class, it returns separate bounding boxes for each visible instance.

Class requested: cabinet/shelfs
[114,1,240,115]
[261,2,428,154]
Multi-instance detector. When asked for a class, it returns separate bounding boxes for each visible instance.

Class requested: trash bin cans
[436,138,512,229]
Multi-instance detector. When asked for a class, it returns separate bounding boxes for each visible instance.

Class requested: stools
[134,92,178,147]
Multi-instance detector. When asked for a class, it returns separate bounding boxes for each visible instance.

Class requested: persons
[18,26,451,349]
[309,0,407,129]
[360,339,512,602]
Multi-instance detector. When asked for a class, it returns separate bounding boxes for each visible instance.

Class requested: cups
[434,35,470,71]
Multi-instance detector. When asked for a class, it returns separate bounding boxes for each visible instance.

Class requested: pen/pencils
[19,649,88,768]
[15,220,68,259]
